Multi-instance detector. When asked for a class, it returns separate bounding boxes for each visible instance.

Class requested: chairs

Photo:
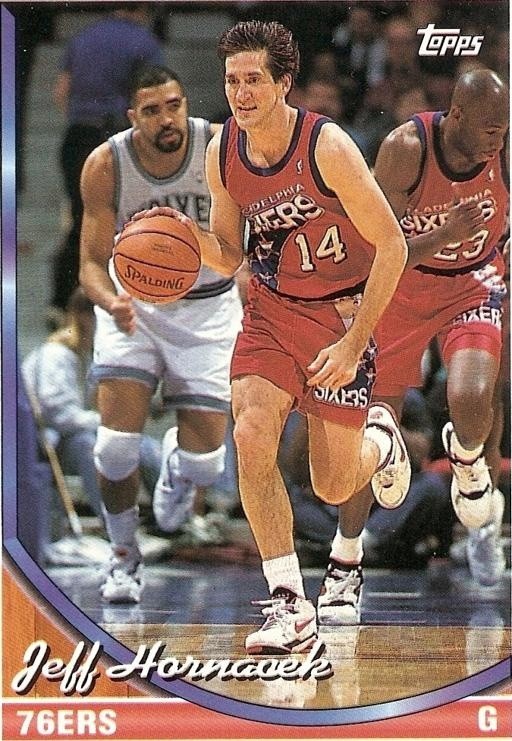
[21,368,83,538]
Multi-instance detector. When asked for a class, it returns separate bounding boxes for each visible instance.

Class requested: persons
[1,0,509,658]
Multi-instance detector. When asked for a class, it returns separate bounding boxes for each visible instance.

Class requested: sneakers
[98,547,148,605]
[151,426,198,532]
[363,400,412,511]
[315,552,366,626]
[439,421,487,525]
[465,491,507,586]
[241,599,318,653]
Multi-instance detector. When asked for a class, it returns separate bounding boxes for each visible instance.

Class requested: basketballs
[113,218,199,304]
